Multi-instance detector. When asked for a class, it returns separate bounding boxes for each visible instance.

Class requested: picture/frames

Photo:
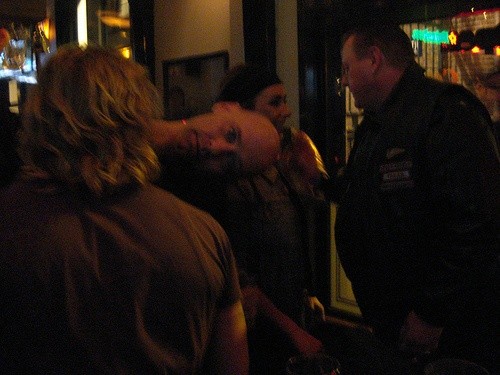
[162,50,231,124]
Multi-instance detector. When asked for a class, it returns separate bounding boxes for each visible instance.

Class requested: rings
[422,349,430,356]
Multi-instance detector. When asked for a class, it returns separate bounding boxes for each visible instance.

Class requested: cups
[286,353,339,375]
[422,359,491,375]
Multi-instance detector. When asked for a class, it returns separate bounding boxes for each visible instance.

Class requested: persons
[0,40,328,375]
[328,23,500,375]
